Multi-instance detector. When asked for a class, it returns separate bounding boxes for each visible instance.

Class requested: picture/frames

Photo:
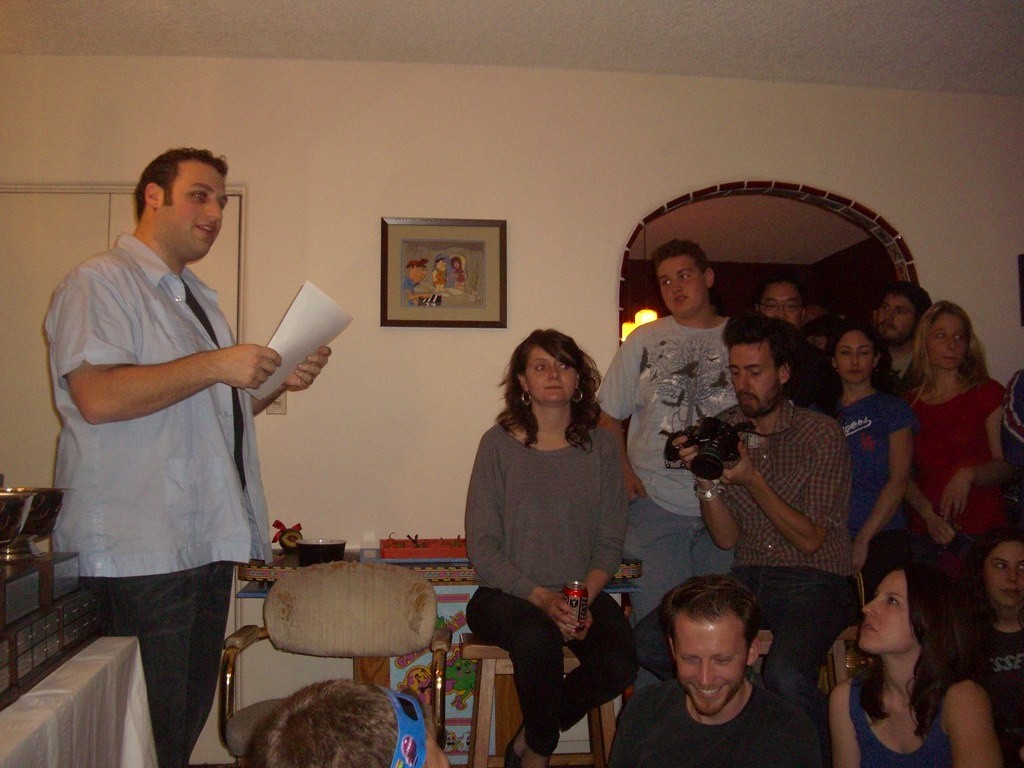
[380,216,507,329]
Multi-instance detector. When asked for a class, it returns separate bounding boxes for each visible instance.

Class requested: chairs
[218,559,453,768]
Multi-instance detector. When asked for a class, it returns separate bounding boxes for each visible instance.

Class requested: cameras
[683,417,743,480]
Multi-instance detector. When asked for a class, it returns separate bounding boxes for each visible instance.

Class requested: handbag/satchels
[825,570,882,690]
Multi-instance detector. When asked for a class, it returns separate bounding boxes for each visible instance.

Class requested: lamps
[621,225,658,342]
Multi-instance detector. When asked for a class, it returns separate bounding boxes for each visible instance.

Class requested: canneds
[562,582,589,633]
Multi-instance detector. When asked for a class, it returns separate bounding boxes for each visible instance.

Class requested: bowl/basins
[294,538,348,566]
[0,487,66,559]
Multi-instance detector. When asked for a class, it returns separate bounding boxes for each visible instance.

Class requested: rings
[553,618,560,623]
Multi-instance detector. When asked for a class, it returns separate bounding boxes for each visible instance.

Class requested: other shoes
[519,753,549,768]
[504,718,528,768]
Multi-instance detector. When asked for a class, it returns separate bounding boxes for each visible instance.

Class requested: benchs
[458,629,859,768]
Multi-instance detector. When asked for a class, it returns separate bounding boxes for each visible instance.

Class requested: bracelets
[693,478,727,501]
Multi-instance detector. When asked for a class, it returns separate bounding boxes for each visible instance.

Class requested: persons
[246,679,450,768]
[828,564,1003,768]
[465,330,641,768]
[606,579,822,768]
[968,527,1024,768]
[44,148,331,768]
[595,238,1024,721]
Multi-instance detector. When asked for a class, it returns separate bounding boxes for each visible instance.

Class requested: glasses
[757,298,802,313]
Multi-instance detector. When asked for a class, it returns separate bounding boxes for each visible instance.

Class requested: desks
[235,555,642,768]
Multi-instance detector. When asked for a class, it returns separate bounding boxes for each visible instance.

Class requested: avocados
[278,529,304,552]
